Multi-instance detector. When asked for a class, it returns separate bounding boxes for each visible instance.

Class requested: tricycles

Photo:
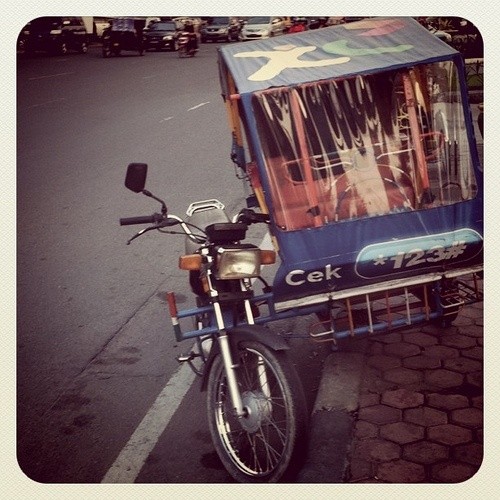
[218,13,483,346]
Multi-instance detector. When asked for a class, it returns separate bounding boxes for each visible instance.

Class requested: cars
[202,17,243,42]
[428,24,452,43]
[145,20,185,50]
[238,18,285,41]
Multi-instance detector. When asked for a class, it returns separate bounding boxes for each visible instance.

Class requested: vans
[28,15,87,55]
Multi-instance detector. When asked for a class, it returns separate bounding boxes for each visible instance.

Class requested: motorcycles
[102,18,148,58]
[176,31,200,57]
[116,160,305,485]
